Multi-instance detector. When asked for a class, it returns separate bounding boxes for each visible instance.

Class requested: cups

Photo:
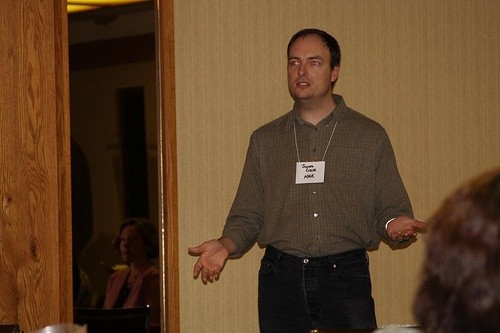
[34,324,88,333]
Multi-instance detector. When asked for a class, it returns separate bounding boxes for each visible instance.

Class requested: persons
[105,219,160,333]
[413,169,500,333]
[70,137,93,307]
[188,29,426,333]
